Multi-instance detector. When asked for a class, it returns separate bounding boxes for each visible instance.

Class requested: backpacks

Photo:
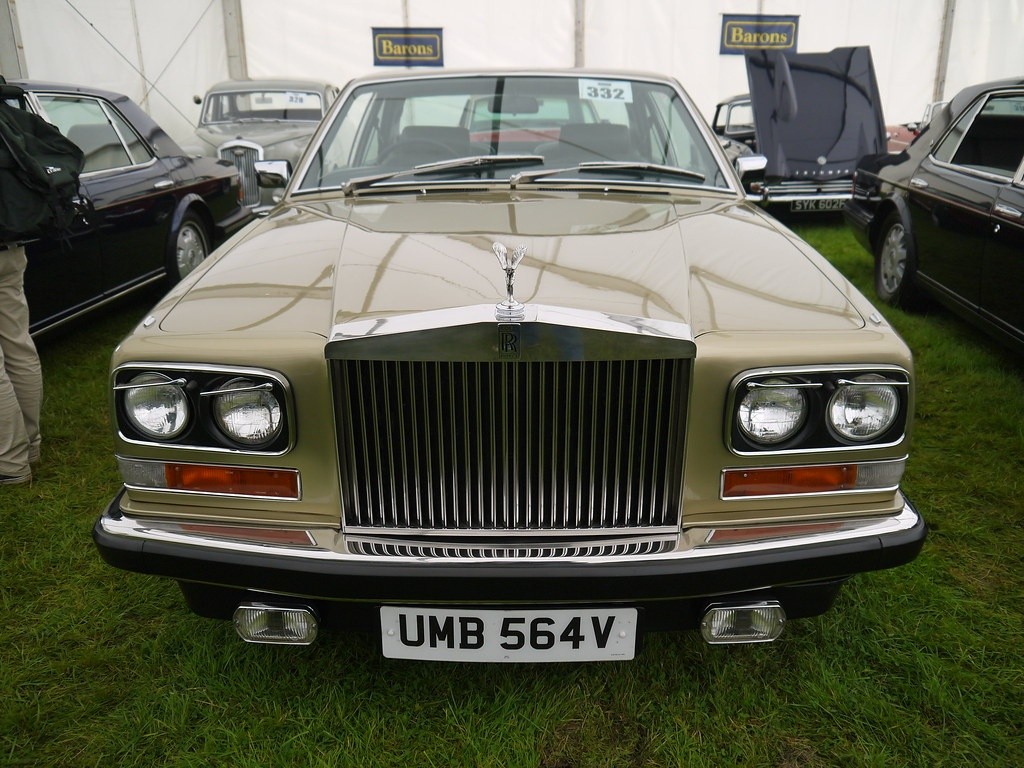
[0,74,96,243]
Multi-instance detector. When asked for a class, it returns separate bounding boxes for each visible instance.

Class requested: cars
[843,79,1024,358]
[178,80,357,217]
[690,45,888,213]
[93,68,926,662]
[4,81,254,338]
[460,93,610,151]
[886,97,1024,154]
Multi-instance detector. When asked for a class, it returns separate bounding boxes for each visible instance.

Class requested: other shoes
[29,455,40,464]
[0,474,33,485]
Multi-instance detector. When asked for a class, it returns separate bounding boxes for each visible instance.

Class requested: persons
[0,75,45,486]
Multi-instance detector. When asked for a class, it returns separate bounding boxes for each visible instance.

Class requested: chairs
[551,122,644,182]
[394,125,470,178]
